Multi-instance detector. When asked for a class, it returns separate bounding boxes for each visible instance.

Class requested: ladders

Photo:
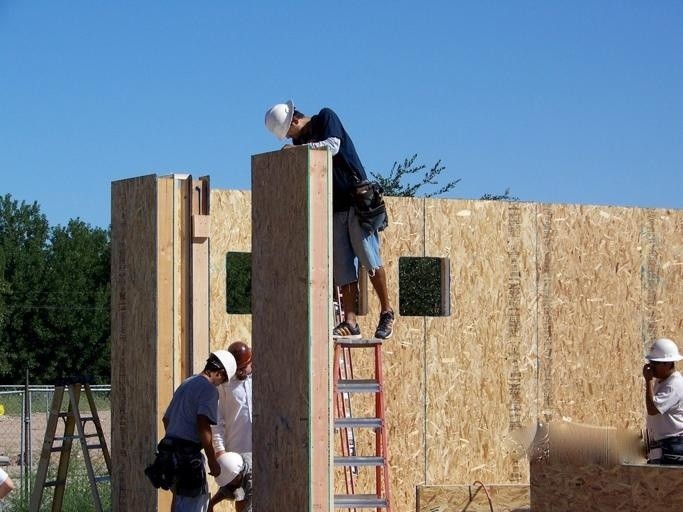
[30,376,111,512]
[333,337,390,512]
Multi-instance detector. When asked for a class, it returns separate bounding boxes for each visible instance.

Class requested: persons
[213,341,253,512]
[0,466,14,502]
[641,338,682,466]
[144,350,238,512]
[206,449,253,512]
[263,101,395,343]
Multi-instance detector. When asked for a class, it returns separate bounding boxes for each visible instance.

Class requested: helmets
[211,350,237,384]
[265,99,294,141]
[644,338,683,363]
[228,342,252,370]
[214,452,244,488]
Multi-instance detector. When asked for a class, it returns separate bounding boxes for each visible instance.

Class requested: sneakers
[332,321,362,340]
[375,307,396,339]
